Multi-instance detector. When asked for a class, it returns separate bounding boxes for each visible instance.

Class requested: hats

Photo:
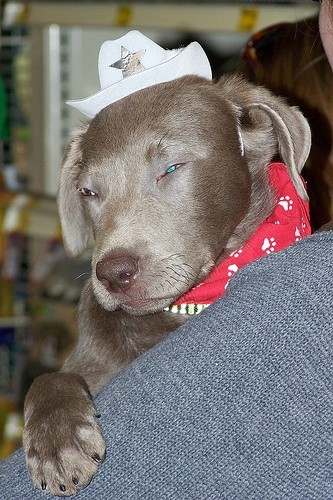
[64,30,214,119]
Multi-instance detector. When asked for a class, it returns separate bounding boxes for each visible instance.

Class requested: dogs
[22,26,310,496]
